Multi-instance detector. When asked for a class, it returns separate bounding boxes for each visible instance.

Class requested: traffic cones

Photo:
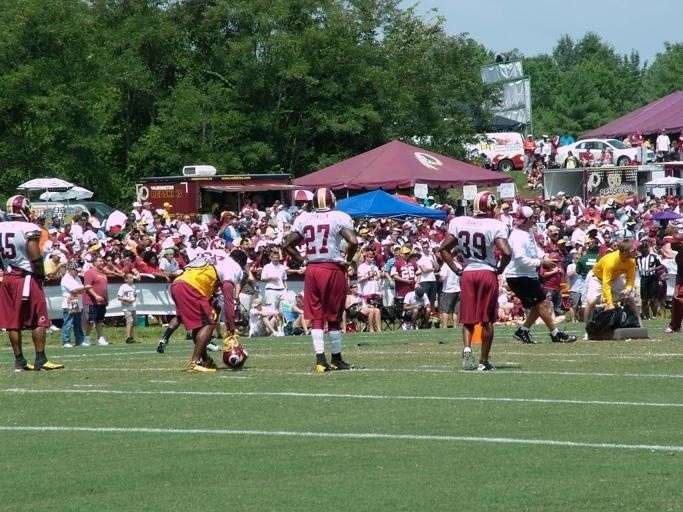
[468,322,483,345]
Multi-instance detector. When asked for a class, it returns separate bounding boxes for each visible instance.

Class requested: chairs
[348,296,411,332]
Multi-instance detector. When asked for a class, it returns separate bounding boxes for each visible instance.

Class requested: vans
[29,200,131,225]
[460,133,526,173]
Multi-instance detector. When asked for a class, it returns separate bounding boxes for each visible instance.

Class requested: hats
[415,287,424,297]
[251,298,262,306]
[358,217,445,258]
[37,202,180,272]
[500,191,676,249]
[190,207,274,250]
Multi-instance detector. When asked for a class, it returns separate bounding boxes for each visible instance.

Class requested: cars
[555,137,656,166]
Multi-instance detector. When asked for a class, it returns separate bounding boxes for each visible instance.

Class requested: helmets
[472,191,497,218]
[6,195,32,222]
[223,334,248,369]
[314,188,336,212]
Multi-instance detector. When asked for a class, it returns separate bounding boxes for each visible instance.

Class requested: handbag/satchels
[585,304,640,340]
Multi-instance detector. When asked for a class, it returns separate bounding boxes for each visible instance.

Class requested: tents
[644,175,682,194]
[336,189,447,221]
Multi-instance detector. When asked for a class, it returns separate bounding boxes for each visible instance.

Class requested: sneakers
[157,336,167,353]
[664,327,673,333]
[126,337,136,343]
[61,337,109,348]
[477,360,496,370]
[14,362,34,372]
[186,358,217,373]
[33,361,64,370]
[272,331,285,336]
[462,346,474,370]
[206,342,219,351]
[512,327,535,343]
[310,363,338,372]
[331,361,355,369]
[549,332,577,343]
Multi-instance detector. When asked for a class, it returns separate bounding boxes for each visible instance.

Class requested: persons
[2,195,65,373]
[583,239,642,342]
[503,206,577,343]
[171,250,247,372]
[249,298,285,336]
[281,188,357,373]
[82,256,109,345]
[117,272,137,343]
[439,190,512,370]
[61,262,93,347]
[156,268,250,353]
[0,191,682,336]
[439,247,463,328]
[522,129,680,190]
